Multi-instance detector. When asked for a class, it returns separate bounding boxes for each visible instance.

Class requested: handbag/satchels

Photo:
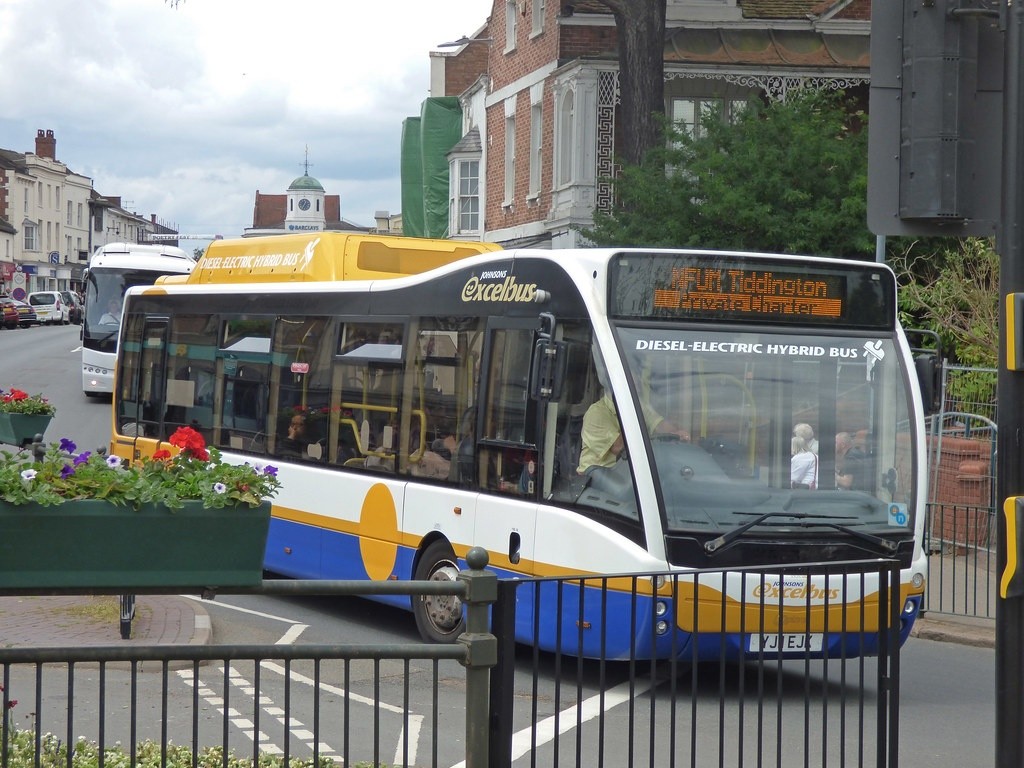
[792,481,811,489]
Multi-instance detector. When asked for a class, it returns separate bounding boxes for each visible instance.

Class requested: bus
[71,241,198,400]
[109,229,944,666]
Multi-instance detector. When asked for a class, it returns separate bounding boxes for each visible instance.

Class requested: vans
[27,291,70,326]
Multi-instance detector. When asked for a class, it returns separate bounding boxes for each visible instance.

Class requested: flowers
[0,384,56,418]
[0,425,282,512]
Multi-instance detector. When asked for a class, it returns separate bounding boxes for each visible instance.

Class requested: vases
[0,498,272,594]
[0,414,54,445]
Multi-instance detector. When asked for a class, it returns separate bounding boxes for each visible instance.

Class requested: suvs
[60,291,85,325]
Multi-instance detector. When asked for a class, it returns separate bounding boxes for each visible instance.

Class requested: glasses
[289,423,304,428]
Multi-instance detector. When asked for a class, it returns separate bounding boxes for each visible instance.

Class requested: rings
[686,436,688,437]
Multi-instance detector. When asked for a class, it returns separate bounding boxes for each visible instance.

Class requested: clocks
[298,199,310,210]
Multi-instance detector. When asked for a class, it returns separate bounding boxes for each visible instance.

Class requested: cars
[12,299,37,329]
[0,295,19,330]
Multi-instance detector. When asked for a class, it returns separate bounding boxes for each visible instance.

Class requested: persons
[442,412,475,456]
[794,423,819,453]
[78,292,82,298]
[576,352,691,475]
[98,301,121,325]
[6,287,13,295]
[277,414,321,453]
[835,433,864,490]
[790,437,818,489]
[366,414,419,471]
